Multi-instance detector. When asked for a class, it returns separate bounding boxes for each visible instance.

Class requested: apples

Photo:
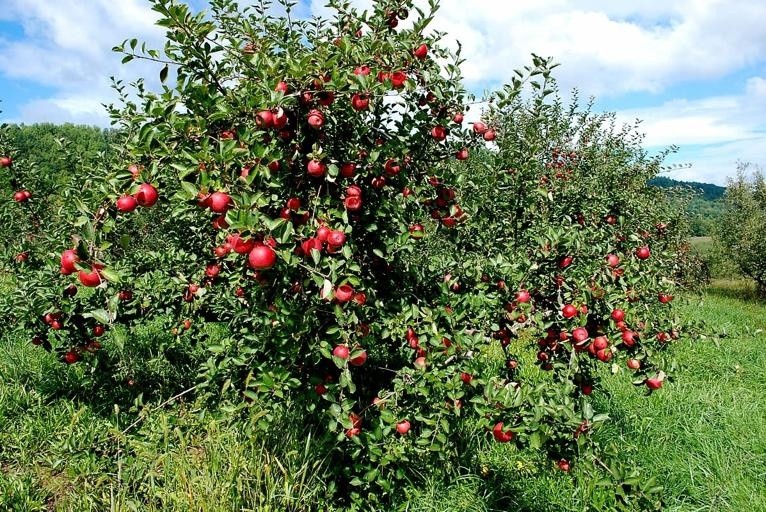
[0,7,679,472]
